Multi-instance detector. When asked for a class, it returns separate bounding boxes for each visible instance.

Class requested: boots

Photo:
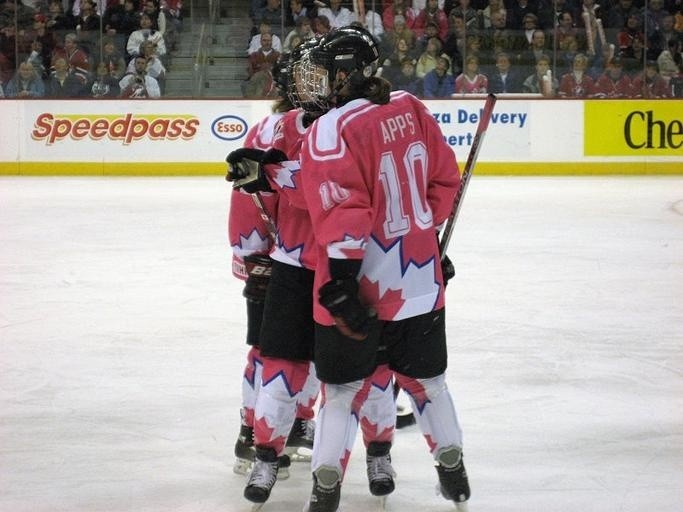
[285,417,315,449]
[234,409,291,467]
[366,442,395,496]
[435,445,470,503]
[247,445,278,503]
[308,466,343,512]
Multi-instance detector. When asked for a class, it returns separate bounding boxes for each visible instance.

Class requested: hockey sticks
[394,94,496,428]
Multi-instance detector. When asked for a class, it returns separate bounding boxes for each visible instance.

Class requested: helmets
[273,25,380,123]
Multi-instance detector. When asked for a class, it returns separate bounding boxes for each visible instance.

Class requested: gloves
[224,145,288,197]
[321,276,369,344]
[434,233,456,286]
[241,255,273,307]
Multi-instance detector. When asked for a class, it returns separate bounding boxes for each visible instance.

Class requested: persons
[225,42,316,463]
[217,32,401,502]
[242,1,683,103]
[1,0,186,99]
[293,20,473,511]
